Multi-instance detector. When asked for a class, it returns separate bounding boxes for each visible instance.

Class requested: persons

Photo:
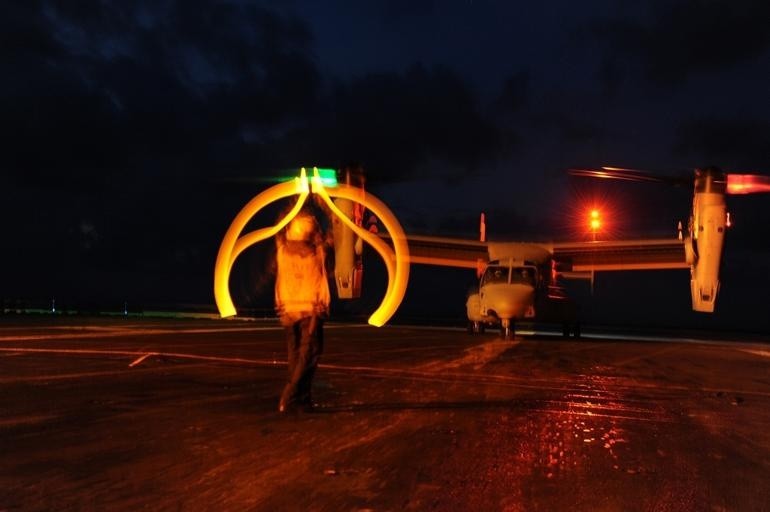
[494,271,503,281]
[265,213,333,418]
[518,269,531,281]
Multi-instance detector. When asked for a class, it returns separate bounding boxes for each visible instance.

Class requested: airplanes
[316,160,770,339]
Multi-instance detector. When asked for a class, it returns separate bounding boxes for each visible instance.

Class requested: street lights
[587,207,603,296]
